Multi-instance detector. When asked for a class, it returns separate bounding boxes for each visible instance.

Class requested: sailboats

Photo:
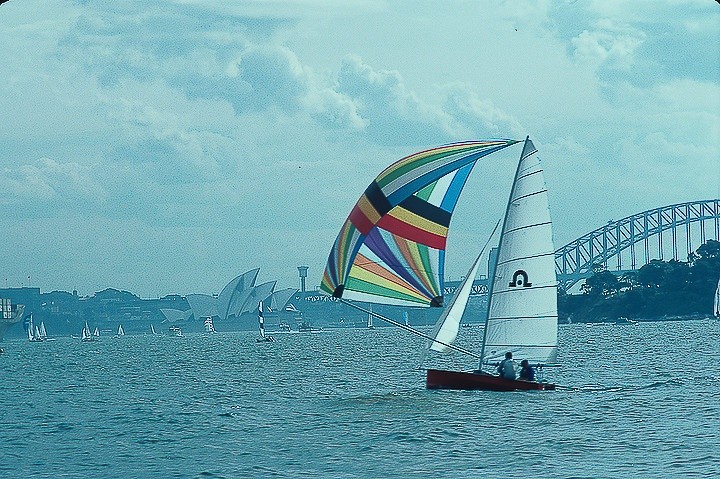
[319,135,562,391]
[80,320,104,342]
[204,318,220,337]
[714,279,720,321]
[23,311,58,342]
[112,324,125,339]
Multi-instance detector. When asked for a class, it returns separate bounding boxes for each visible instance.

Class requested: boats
[150,324,163,337]
[168,325,184,338]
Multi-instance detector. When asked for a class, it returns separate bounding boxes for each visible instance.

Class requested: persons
[517,360,534,381]
[497,352,519,381]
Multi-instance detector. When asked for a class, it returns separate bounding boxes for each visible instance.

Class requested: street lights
[28,276,30,288]
[6,278,7,289]
